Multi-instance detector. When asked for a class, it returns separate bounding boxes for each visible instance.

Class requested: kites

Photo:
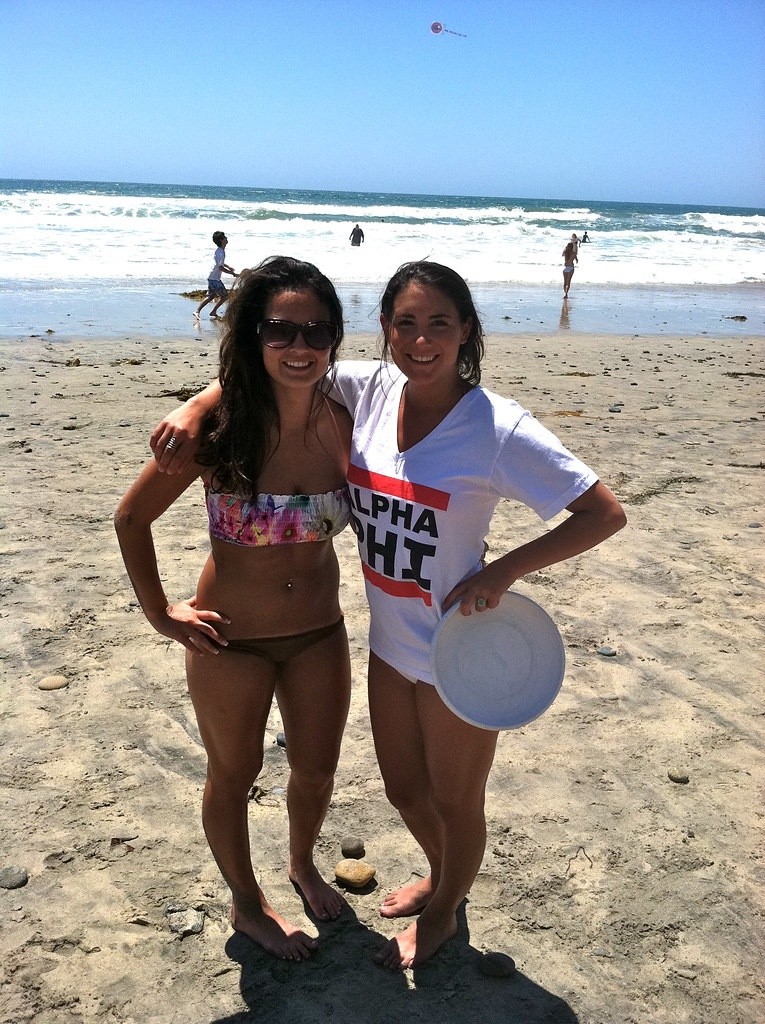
[431,22,466,38]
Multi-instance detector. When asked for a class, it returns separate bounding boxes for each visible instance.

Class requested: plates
[430,593,568,732]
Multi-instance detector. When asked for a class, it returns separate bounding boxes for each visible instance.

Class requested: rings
[477,598,485,606]
[167,438,178,450]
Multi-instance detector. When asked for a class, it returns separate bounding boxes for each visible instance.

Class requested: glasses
[255,319,340,350]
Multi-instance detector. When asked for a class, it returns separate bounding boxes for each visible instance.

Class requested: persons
[562,243,578,298]
[192,231,239,321]
[150,262,627,969]
[349,224,364,246]
[582,232,590,243]
[571,234,581,255]
[114,254,488,958]
